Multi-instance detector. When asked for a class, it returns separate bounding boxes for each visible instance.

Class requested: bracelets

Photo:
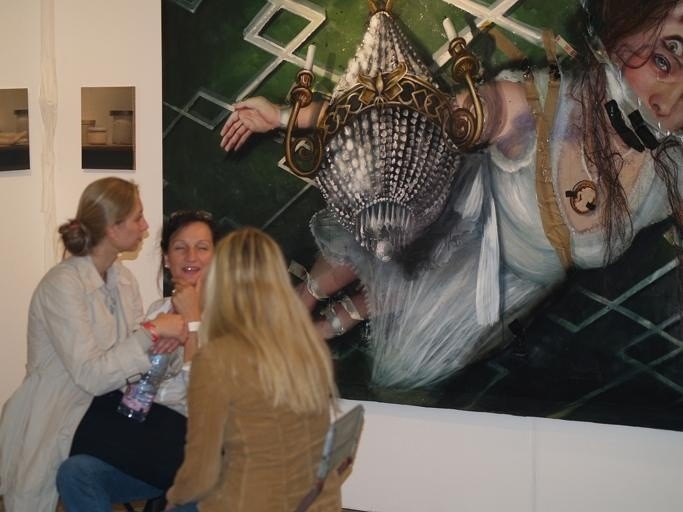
[188,321,200,333]
[277,105,297,131]
[142,320,159,342]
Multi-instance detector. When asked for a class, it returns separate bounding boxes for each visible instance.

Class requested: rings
[170,288,176,294]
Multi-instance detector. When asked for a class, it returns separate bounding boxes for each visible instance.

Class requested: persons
[1,178,189,510]
[201,1,683,350]
[165,227,344,511]
[54,206,217,512]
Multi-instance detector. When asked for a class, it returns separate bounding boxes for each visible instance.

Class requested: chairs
[295,403,365,512]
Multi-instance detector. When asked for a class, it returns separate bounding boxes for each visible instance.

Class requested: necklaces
[566,77,637,215]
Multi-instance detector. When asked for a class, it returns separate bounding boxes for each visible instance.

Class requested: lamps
[285,1,485,265]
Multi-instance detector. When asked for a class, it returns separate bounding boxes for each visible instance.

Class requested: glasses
[170,209,213,220]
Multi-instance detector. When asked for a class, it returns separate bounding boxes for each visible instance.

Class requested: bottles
[118,348,169,424]
